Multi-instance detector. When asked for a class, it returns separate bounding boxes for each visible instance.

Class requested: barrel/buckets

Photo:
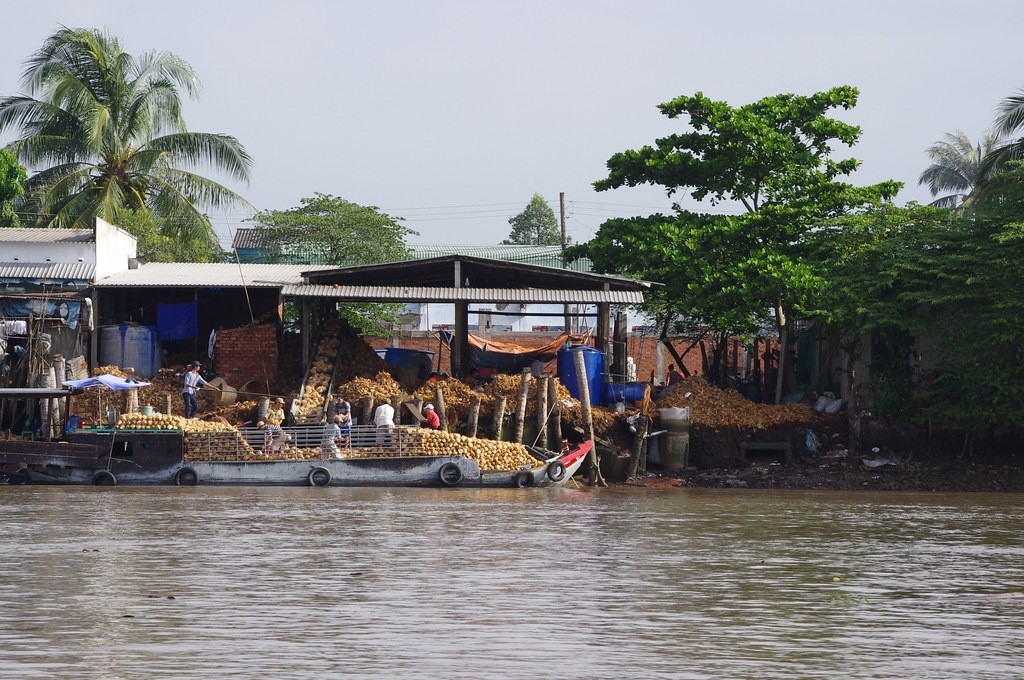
[385,347,435,394]
[557,346,604,406]
[604,381,654,404]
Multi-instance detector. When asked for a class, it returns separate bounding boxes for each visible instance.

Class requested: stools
[21,430,34,441]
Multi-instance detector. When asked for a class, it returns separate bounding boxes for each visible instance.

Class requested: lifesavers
[514,469,535,489]
[547,460,566,482]
[307,466,332,487]
[174,466,200,486]
[91,471,117,486]
[438,462,463,487]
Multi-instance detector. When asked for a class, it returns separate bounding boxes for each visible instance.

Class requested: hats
[256,421,265,429]
[383,398,391,405]
[423,404,434,410]
[334,415,343,423]
[191,361,202,367]
[275,398,285,404]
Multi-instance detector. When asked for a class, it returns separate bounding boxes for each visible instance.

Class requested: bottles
[81,421,85,429]
[66,413,79,431]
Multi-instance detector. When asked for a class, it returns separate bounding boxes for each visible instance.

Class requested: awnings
[283,286,644,304]
[94,261,341,286]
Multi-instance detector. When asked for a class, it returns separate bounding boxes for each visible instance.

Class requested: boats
[0,387,595,489]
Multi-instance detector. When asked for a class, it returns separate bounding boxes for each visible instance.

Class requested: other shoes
[258,449,262,455]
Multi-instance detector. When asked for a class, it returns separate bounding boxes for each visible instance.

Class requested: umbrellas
[63,374,151,429]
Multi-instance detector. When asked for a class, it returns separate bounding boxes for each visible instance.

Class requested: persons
[666,363,683,386]
[256,397,287,457]
[333,394,352,450]
[320,415,342,460]
[182,361,220,419]
[374,398,396,454]
[420,404,441,430]
